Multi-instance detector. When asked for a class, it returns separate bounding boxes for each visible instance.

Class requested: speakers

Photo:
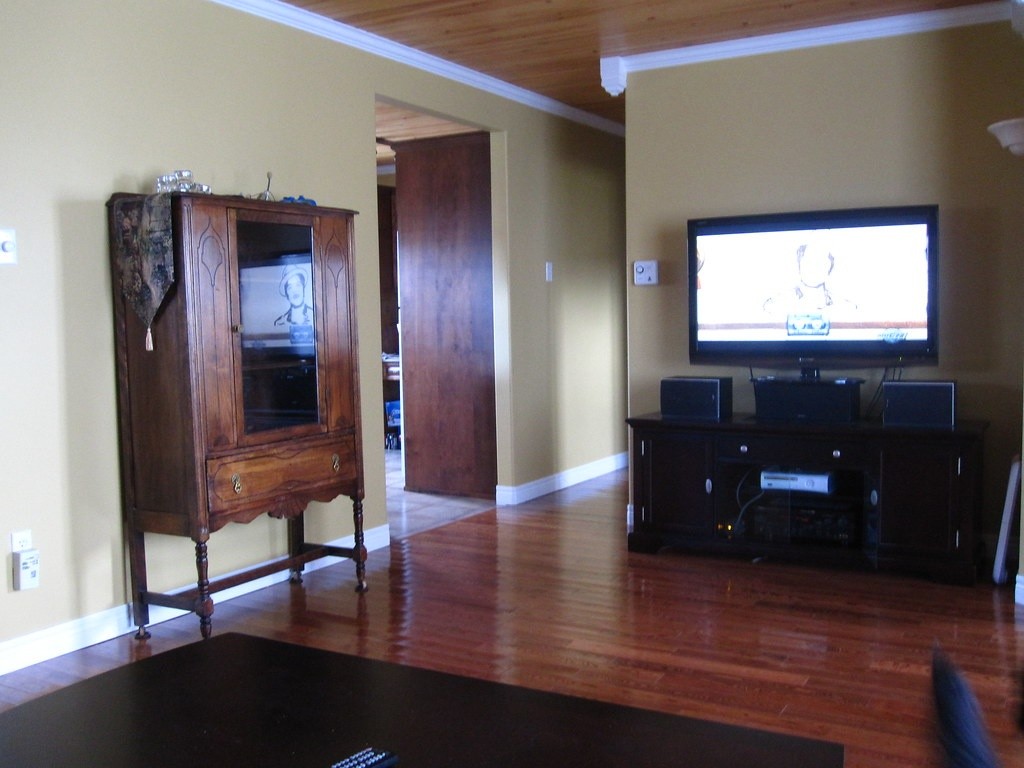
[660,376,734,422]
[754,383,861,425]
[883,381,959,427]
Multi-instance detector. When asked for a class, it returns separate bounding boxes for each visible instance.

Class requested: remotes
[332,746,399,768]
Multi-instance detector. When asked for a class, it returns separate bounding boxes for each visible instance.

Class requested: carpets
[0,632,847,768]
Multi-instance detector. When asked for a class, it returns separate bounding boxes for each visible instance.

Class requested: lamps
[987,118,1024,157]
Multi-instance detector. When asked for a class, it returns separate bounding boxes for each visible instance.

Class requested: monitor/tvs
[687,203,940,384]
[238,253,316,360]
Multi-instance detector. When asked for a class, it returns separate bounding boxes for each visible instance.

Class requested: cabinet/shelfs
[626,410,991,587]
[107,191,369,639]
